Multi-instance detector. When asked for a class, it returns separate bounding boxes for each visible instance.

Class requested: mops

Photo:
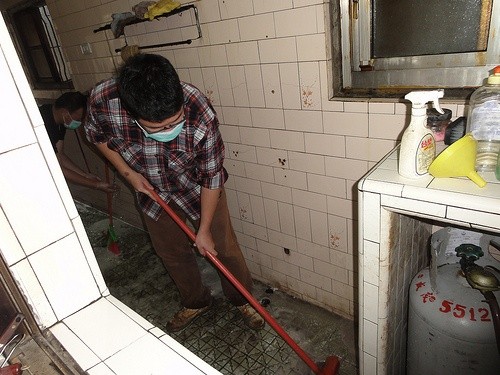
[148,187,338,374]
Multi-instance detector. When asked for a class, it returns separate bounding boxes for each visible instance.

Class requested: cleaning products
[399,88,446,176]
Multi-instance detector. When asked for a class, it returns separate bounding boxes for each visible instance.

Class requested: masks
[134,120,186,142]
[63,114,82,129]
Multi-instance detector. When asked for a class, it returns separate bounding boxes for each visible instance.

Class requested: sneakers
[237,302,265,331]
[166,300,212,332]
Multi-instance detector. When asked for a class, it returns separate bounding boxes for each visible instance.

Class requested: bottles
[465,65,500,184]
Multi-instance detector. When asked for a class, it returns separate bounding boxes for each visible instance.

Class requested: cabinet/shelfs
[93,5,202,52]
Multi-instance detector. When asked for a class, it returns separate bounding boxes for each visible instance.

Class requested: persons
[38,91,115,193]
[83,53,265,332]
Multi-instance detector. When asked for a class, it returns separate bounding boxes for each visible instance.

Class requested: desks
[358,143,500,375]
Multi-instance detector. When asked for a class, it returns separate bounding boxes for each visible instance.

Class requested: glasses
[136,115,185,133]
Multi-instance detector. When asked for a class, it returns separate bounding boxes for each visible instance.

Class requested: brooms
[105,164,119,254]
